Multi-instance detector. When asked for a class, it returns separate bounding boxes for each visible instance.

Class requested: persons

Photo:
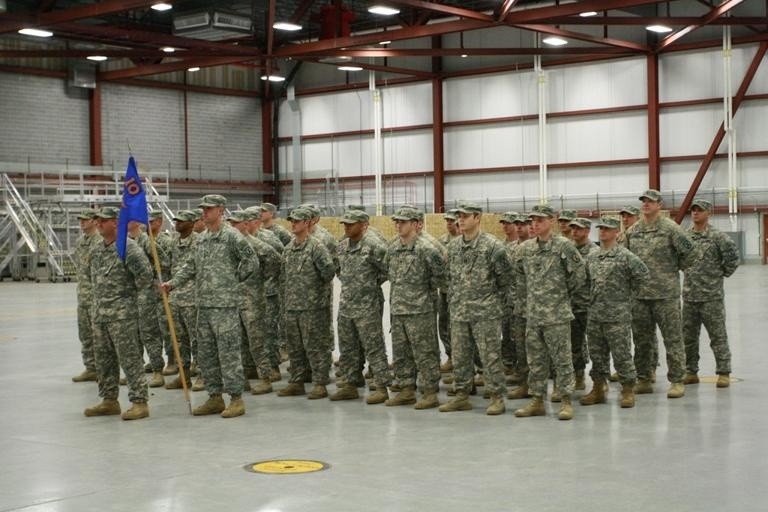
[69,188,698,421]
[680,200,738,387]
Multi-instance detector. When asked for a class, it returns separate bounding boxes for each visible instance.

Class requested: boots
[85,398,121,416]
[620,384,635,408]
[121,402,149,420]
[220,393,245,418]
[366,382,439,409]
[580,376,609,405]
[193,394,224,415]
[249,349,365,400]
[439,357,585,420]
[74,359,205,392]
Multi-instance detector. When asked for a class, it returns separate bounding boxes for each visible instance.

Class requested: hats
[595,217,620,229]
[567,217,591,228]
[529,205,554,217]
[557,210,577,220]
[689,200,712,211]
[389,205,423,221]
[77,202,321,222]
[639,190,662,202]
[499,211,532,223]
[443,203,482,220]
[339,204,369,225]
[618,205,640,215]
[197,194,227,206]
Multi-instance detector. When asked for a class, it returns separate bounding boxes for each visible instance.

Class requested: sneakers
[609,372,618,381]
[684,374,699,384]
[632,381,652,394]
[667,383,684,398]
[717,375,729,387]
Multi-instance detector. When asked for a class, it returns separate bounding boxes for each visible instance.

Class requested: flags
[115,159,149,262]
